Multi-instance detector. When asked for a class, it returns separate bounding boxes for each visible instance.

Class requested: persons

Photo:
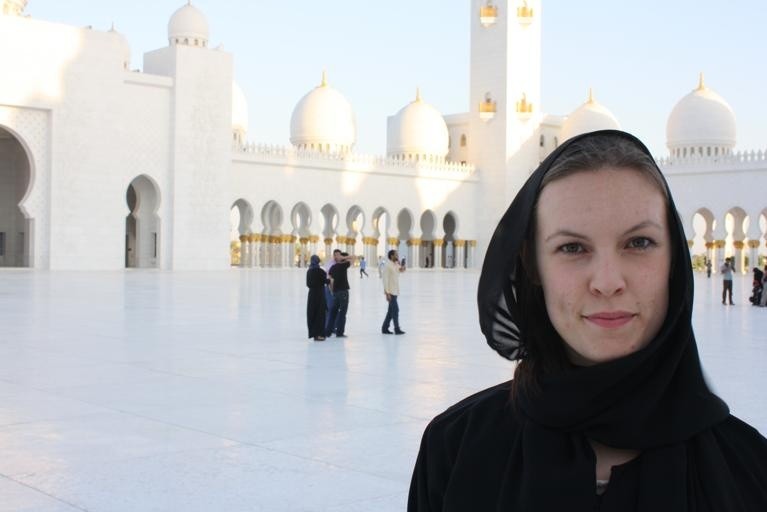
[306,250,350,340]
[406,128,767,512]
[381,250,406,335]
[748,265,767,307]
[358,256,368,279]
[720,257,736,305]
[705,259,712,278]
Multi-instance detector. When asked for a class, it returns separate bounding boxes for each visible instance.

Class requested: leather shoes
[382,329,393,334]
[395,330,405,334]
